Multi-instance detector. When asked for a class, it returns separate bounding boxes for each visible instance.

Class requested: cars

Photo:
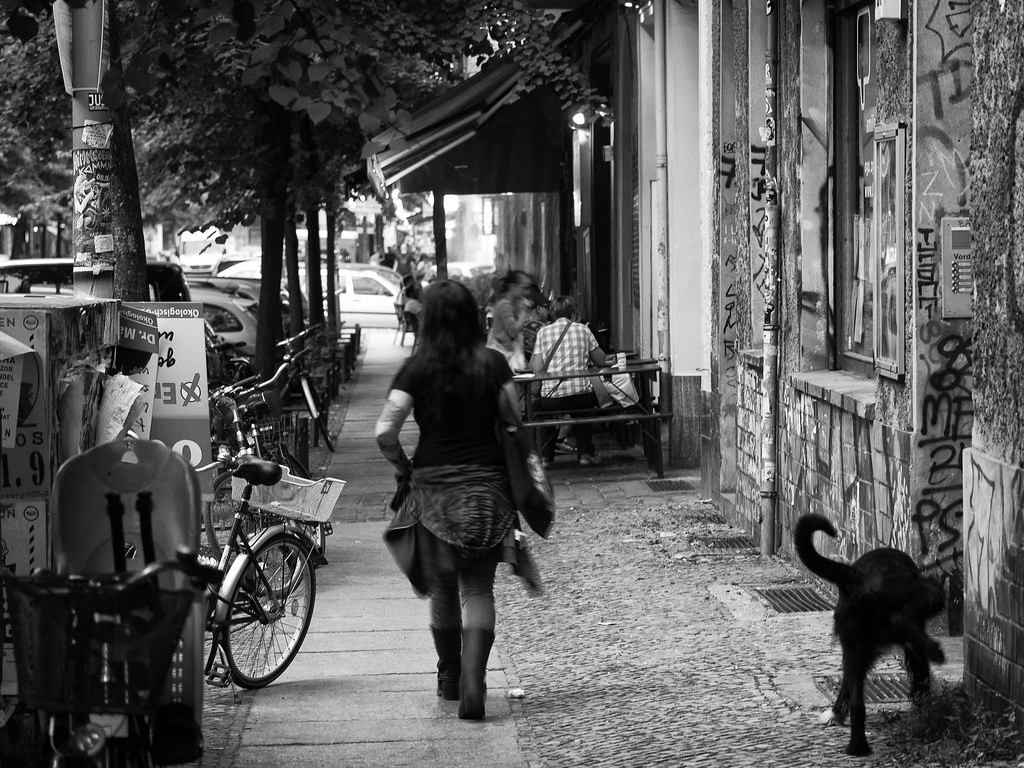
[190,287,258,355]
[187,274,308,338]
[204,320,236,384]
[220,255,285,291]
[287,267,405,333]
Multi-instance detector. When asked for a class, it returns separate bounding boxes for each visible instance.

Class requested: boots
[457,628,495,720]
[429,624,462,700]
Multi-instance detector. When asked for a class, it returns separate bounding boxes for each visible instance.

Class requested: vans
[282,226,366,265]
[0,258,192,304]
[179,224,226,276]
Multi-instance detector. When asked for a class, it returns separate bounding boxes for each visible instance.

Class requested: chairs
[507,345,673,482]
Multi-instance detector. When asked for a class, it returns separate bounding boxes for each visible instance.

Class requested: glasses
[540,304,549,310]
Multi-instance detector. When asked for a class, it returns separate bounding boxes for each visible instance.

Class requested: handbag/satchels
[503,427,554,541]
[530,380,541,396]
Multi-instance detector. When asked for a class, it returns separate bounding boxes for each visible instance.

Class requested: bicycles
[206,373,319,600]
[220,346,331,560]
[194,446,328,693]
[203,323,338,455]
[0,545,222,767]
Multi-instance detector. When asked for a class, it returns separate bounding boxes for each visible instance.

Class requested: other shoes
[579,454,602,465]
[555,443,578,455]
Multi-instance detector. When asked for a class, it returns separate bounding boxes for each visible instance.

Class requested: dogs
[794,512,949,758]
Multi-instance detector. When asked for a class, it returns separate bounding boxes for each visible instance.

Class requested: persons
[371,246,432,281]
[490,276,531,375]
[376,281,522,713]
[396,276,422,305]
[404,285,425,333]
[531,298,605,470]
